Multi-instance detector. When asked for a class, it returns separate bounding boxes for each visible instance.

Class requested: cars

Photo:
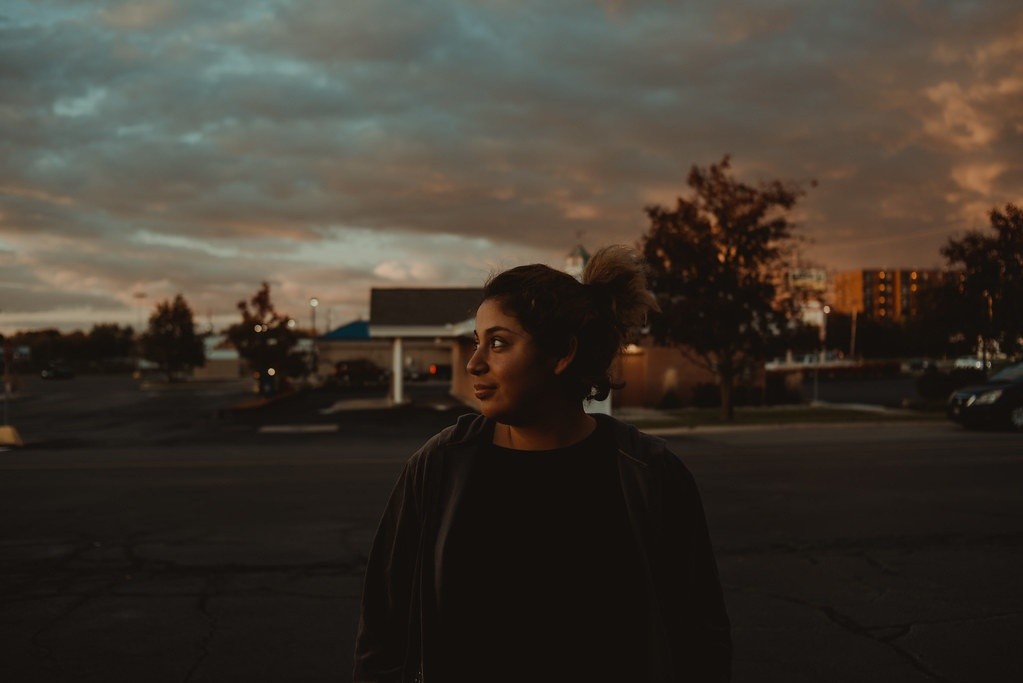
[947,361,1023,433]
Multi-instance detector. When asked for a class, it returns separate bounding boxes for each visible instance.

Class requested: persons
[353,264,734,683]
